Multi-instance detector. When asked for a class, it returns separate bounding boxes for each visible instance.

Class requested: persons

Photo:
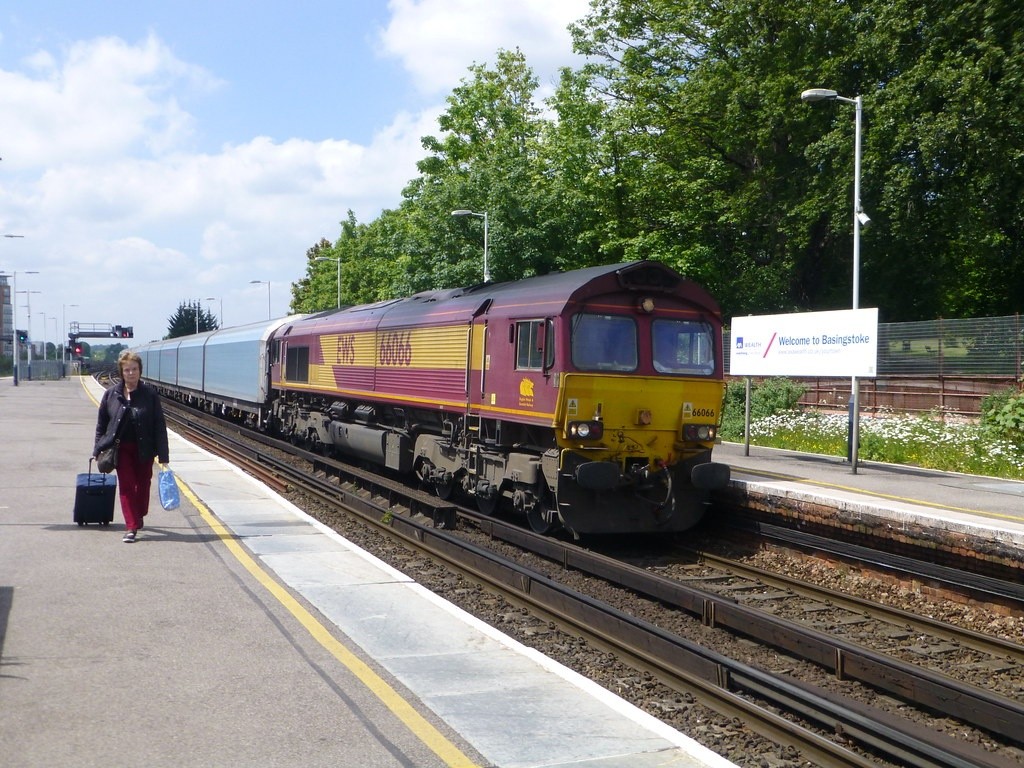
[93,352,171,543]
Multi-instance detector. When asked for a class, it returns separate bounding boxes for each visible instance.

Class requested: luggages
[73,457,116,527]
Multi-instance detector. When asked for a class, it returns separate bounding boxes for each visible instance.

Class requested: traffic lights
[122,333,127,337]
[76,347,81,352]
[20,335,26,343]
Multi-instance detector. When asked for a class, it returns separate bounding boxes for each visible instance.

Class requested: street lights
[801,88,863,474]
[0,234,76,386]
[250,281,271,320]
[206,297,224,327]
[184,306,199,334]
[315,257,341,308]
[452,210,489,282]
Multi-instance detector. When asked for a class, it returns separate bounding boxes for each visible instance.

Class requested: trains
[120,259,731,540]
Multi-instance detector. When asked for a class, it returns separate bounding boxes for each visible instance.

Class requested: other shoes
[122,529,137,542]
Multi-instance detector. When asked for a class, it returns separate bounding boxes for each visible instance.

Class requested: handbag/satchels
[97,446,119,474]
[158,463,180,511]
